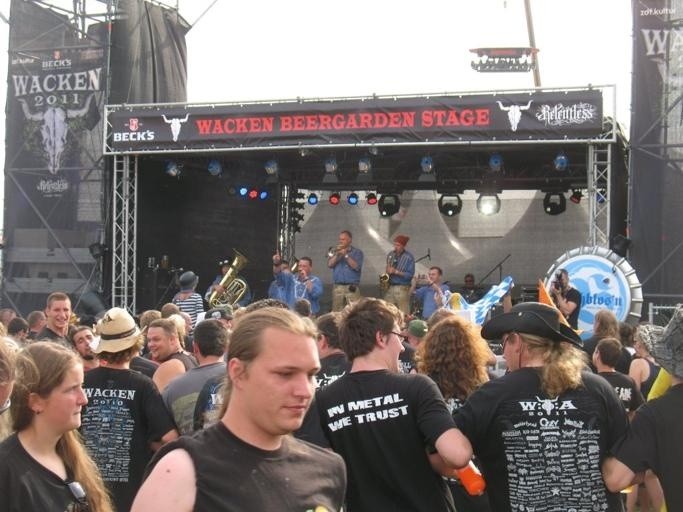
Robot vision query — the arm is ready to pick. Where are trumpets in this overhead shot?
[326,245,346,257]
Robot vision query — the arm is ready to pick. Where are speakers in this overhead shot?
[142,269,184,311]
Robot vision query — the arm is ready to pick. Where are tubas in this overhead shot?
[208,248,250,308]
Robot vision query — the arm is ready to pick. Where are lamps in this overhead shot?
[472,182,505,216]
[166,150,569,180]
[436,184,463,217]
[377,184,403,216]
[568,185,608,206]
[294,186,376,205]
[541,185,569,216]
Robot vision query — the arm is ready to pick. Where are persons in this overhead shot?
[271,245,323,315]
[550,269,580,330]
[499,276,512,314]
[267,261,292,303]
[294,291,683,511]
[130,307,346,511]
[409,266,449,322]
[385,236,414,313]
[204,259,251,307]
[1,291,293,512]
[171,270,203,337]
[460,273,482,304]
[327,230,363,311]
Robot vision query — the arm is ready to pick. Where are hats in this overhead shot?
[407,319,428,338]
[481,302,584,348]
[393,235,409,247]
[638,303,683,379]
[178,271,197,293]
[88,307,148,354]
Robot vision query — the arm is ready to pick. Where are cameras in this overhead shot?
[551,270,562,290]
[418,275,426,278]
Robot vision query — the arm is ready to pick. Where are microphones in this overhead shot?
[428,248,431,260]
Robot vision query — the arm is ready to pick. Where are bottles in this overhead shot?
[456,457,485,497]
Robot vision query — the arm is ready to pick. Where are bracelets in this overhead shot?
[302,276,309,281]
[344,254,348,259]
[272,263,280,267]
[427,281,433,287]
[394,270,398,275]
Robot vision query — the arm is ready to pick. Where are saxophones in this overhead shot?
[379,251,397,291]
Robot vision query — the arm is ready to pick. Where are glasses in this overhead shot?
[382,330,408,344]
